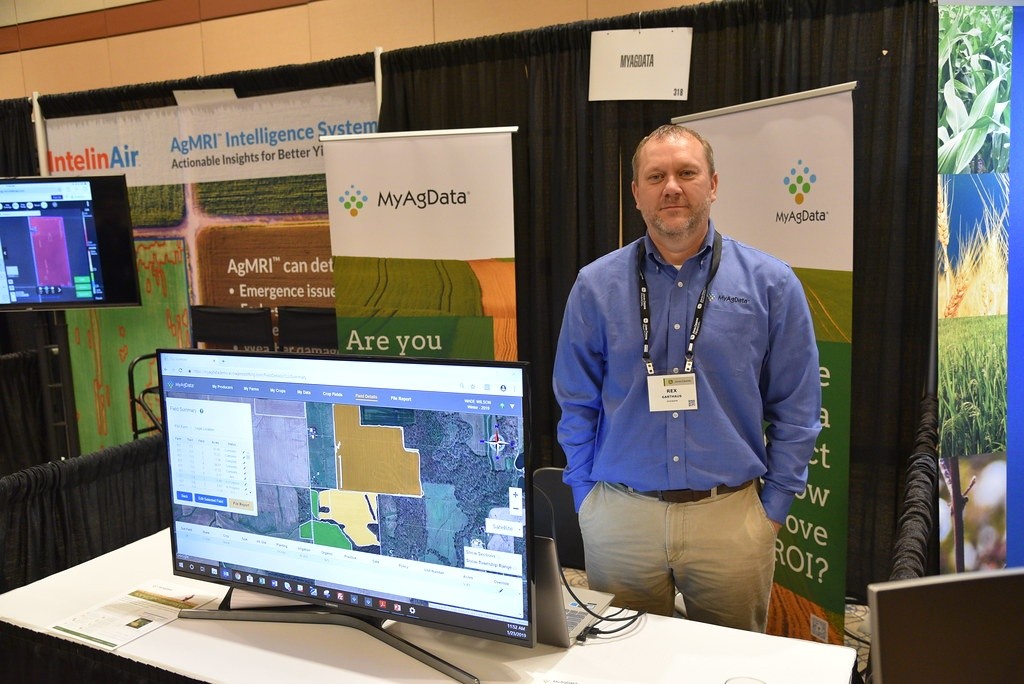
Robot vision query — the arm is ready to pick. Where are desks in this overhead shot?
[0,527,859,684]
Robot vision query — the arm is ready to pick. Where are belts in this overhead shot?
[621,479,755,504]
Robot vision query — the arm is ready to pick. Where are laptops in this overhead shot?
[531,536,616,651]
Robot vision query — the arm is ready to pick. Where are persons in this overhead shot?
[553,124,820,633]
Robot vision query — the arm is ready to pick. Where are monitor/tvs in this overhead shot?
[870,567,1024,684]
[0,175,141,313]
[154,345,534,684]
